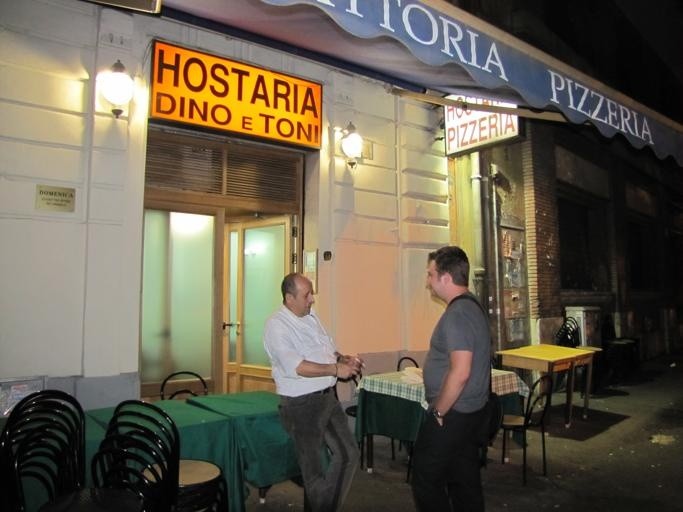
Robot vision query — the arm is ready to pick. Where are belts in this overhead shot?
[312,387,330,395]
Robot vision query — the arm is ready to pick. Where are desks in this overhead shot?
[494,343,596,433]
[186,391,327,504]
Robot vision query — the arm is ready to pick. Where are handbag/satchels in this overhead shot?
[484,390,503,445]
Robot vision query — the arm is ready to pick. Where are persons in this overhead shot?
[260,271,364,511]
[404,245,495,511]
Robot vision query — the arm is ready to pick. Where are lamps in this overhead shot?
[341,121,364,168]
[101,59,135,119]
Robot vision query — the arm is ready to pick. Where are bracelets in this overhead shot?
[333,363,338,377]
[336,354,342,364]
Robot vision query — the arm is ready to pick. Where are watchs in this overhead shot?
[432,407,445,421]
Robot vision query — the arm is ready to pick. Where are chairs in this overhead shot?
[0,371,228,512]
[483,375,553,485]
[554,316,604,398]
[335,356,420,482]
